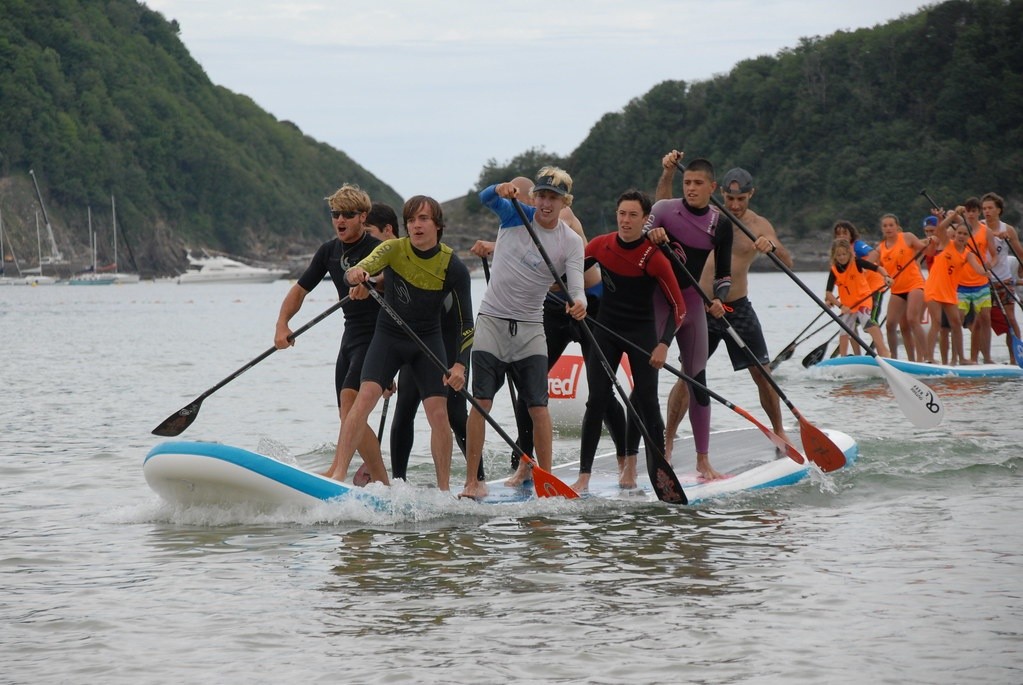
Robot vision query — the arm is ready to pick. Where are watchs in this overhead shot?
[768,241,777,252]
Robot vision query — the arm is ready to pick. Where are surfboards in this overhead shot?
[138,426,857,520]
[815,355,1023,380]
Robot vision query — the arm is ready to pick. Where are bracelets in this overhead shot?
[885,275,891,278]
[839,304,844,308]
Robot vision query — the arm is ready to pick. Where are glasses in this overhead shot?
[329,210,360,219]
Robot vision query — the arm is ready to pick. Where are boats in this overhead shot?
[178,248,291,284]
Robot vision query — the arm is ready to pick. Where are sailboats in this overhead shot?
[0,169,141,286]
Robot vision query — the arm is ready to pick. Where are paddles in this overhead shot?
[865,254,925,356]
[1001,236,1023,269]
[511,185,689,505]
[546,292,806,465]
[776,296,839,362]
[919,188,1023,313]
[769,281,890,371]
[150,294,352,438]
[360,272,581,500]
[665,150,947,432]
[955,210,1023,369]
[481,251,492,285]
[802,237,931,370]
[661,240,847,474]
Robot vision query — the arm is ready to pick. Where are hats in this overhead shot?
[533,175,568,196]
[922,216,937,227]
[722,166,754,193]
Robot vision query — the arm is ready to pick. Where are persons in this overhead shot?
[825,192,1023,365]
[275,149,794,501]
